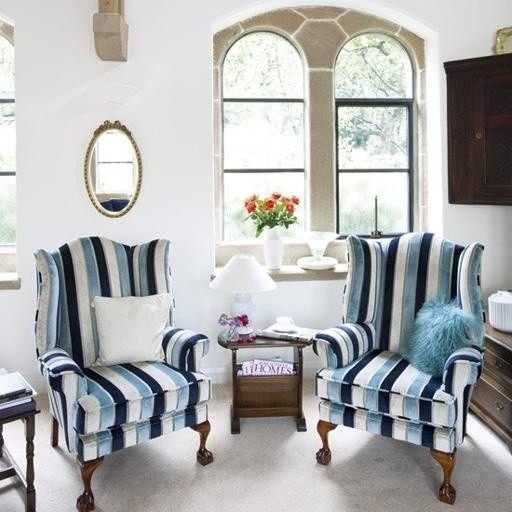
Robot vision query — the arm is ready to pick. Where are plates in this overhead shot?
[273,326,298,333]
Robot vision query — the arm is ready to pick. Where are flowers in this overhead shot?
[241,191,304,239]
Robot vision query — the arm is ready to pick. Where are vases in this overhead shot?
[260,225,285,271]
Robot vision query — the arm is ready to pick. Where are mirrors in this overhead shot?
[83,120,143,219]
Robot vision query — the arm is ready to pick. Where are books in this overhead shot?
[0,371,38,418]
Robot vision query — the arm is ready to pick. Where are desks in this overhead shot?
[0,368,41,511]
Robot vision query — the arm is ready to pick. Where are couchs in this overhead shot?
[310,232,486,504]
[34,237,212,512]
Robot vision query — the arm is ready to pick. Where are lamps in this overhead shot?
[209,254,270,336]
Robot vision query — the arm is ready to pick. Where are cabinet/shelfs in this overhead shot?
[468,303,511,454]
[443,52,512,207]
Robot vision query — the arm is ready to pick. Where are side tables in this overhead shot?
[218,326,314,435]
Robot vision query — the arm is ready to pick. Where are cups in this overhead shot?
[276,316,294,329]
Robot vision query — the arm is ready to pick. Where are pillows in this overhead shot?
[396,293,483,378]
[93,292,171,367]
